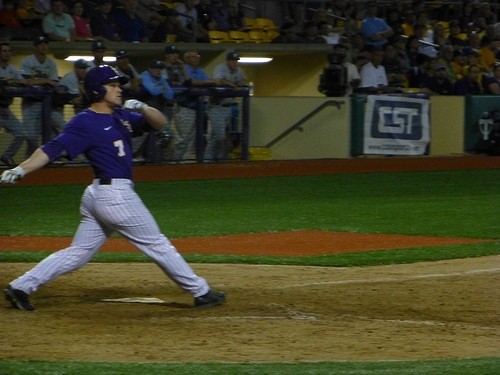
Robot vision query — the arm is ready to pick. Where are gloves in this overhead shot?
[0,166,26,184]
[123,99,148,112]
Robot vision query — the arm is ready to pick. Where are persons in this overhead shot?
[0,65,227,312]
[0,0,500,169]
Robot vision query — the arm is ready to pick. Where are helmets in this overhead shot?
[84,66,129,103]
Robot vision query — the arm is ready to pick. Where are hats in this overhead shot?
[435,65,446,71]
[166,44,181,54]
[148,56,166,69]
[493,62,500,68]
[92,42,107,51]
[34,36,49,45]
[226,52,240,61]
[116,50,129,58]
[74,60,91,68]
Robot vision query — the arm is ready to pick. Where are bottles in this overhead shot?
[249,82,254,96]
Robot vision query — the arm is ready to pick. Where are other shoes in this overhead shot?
[194,289,226,309]
[3,285,34,312]
[0,157,19,166]
